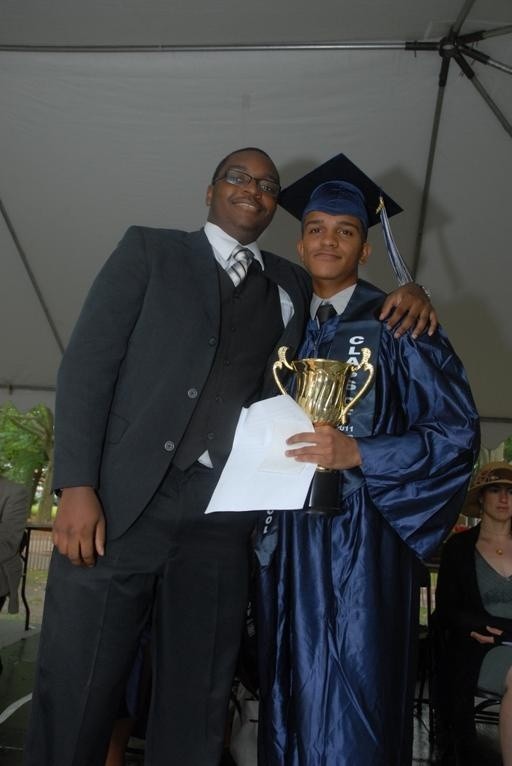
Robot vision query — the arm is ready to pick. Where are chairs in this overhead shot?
[0,527,32,631]
[416,567,503,732]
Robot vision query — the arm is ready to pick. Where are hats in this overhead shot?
[460,462,511,519]
[276,151,405,237]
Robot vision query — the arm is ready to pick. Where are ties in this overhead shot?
[313,302,337,357]
[228,245,249,288]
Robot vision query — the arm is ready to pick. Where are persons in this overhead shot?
[0,472,30,615]
[238,153,480,766]
[425,461,512,766]
[28,147,439,766]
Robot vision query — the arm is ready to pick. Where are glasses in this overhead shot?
[210,168,280,195]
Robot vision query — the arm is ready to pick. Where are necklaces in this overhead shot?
[495,548,504,555]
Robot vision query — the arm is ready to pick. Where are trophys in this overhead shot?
[273,345,376,515]
